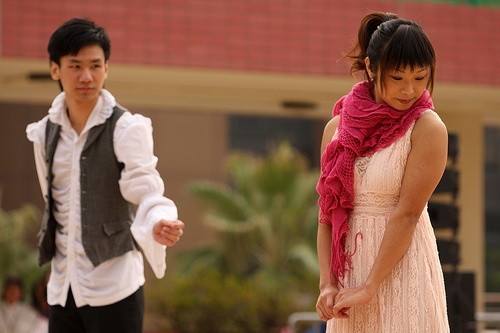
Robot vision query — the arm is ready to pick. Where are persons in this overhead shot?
[0,274,52,333]
[24,16,184,333]
[315,11,451,333]
